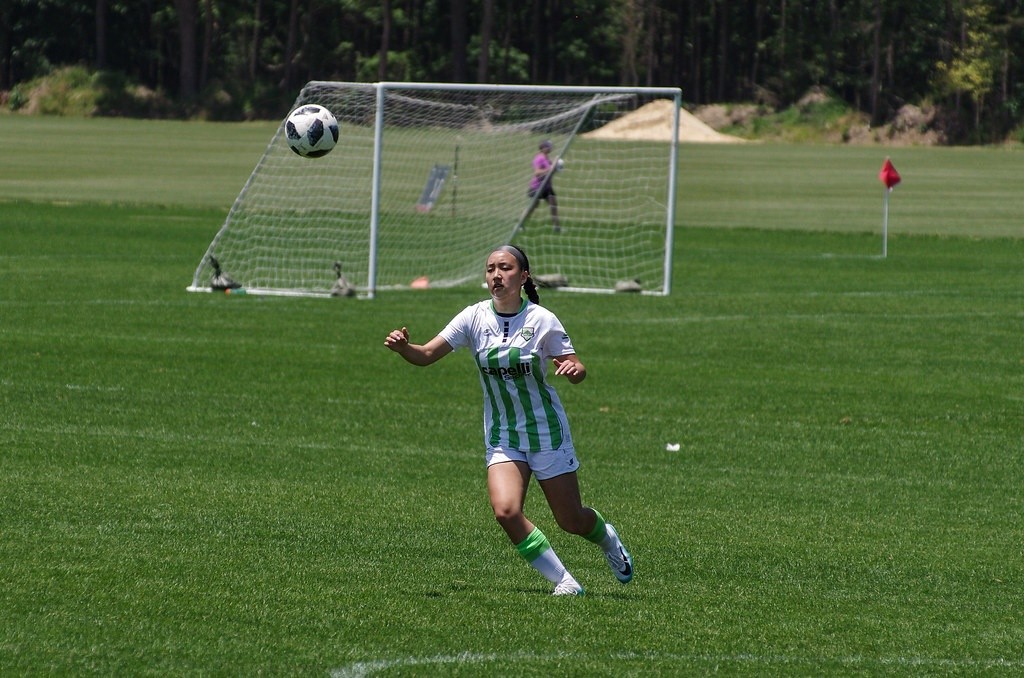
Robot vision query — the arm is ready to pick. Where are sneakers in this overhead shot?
[550,581,585,597]
[599,524,634,584]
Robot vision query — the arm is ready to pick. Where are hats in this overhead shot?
[539,140,553,150]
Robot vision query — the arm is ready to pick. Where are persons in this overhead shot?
[518,140,565,234]
[383,244,633,595]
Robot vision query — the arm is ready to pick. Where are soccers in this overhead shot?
[285,103,339,159]
